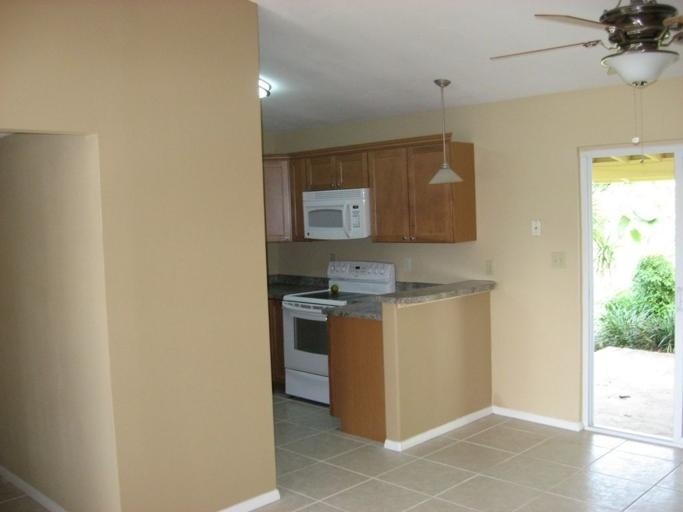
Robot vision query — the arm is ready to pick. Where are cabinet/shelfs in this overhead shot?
[371,142,477,244]
[264,154,300,243]
[306,151,369,191]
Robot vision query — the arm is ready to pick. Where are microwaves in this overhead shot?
[301,187,370,242]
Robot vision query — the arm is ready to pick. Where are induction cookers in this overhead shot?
[282,289,376,307]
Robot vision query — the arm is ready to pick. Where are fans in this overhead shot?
[489,0,683,87]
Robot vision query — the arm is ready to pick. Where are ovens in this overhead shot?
[281,300,333,407]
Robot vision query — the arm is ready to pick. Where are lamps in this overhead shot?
[601,45,679,87]
[256,77,273,100]
[428,78,465,186]
